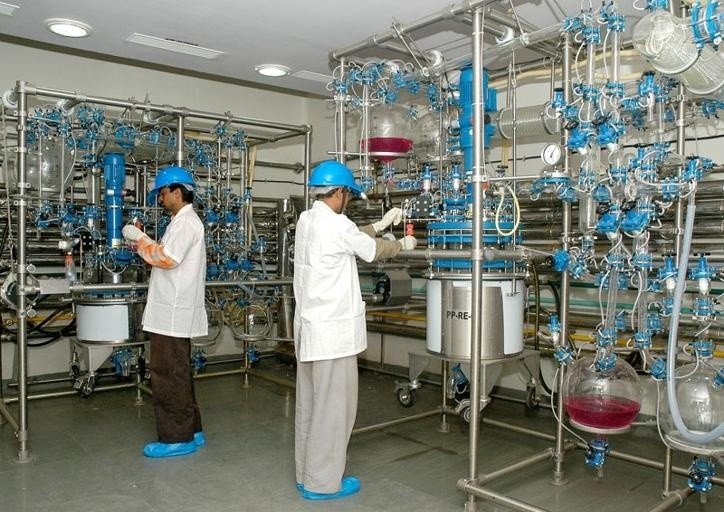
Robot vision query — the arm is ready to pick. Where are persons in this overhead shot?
[122,168,209,457]
[290,164,417,501]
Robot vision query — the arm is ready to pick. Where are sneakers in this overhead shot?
[193,432,208,450]
[141,437,198,458]
[302,475,361,501]
[295,482,303,493]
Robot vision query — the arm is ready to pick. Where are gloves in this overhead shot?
[121,224,146,243]
[371,206,402,234]
[398,235,418,251]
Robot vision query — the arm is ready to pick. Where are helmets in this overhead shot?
[303,159,364,195]
[151,166,197,196]
